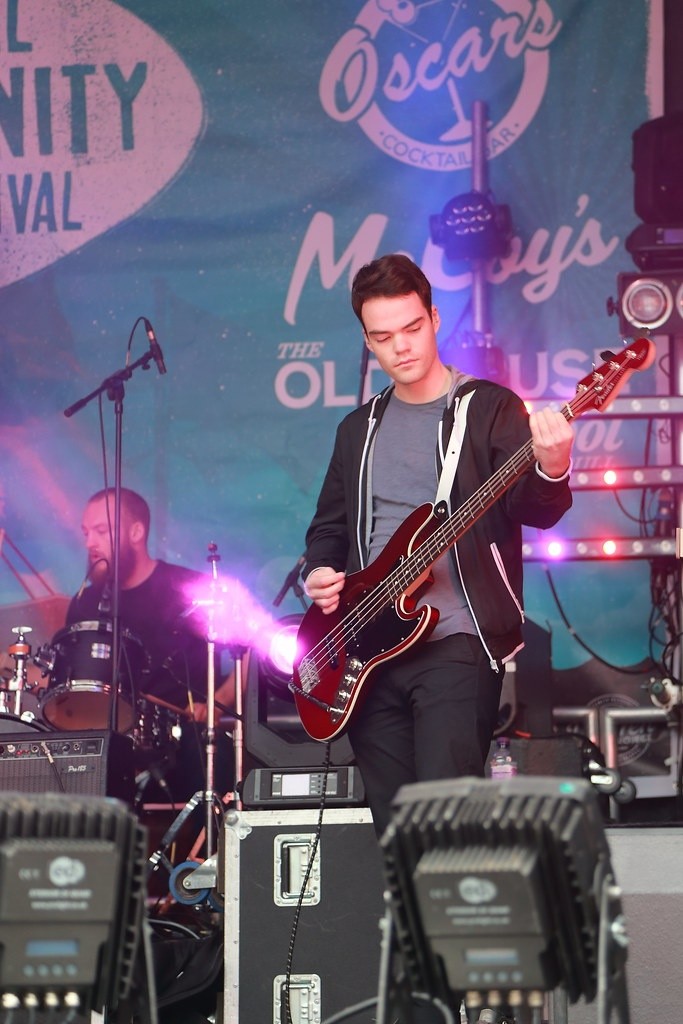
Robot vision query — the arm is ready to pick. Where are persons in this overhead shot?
[301,255,574,840]
[50,487,251,803]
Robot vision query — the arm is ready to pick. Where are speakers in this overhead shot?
[491,617,553,737]
[0,729,135,811]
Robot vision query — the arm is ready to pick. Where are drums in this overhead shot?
[39,618,148,734]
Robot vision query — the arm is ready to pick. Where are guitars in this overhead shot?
[291,334,657,745]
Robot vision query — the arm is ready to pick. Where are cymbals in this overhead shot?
[1,650,50,698]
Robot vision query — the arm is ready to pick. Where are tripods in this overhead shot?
[148,542,245,874]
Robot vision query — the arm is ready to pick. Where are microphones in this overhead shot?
[98,574,113,612]
[273,553,306,607]
[143,319,166,374]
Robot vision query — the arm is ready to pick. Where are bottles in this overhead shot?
[490,737,518,780]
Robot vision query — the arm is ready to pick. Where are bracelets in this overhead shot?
[214,698,226,713]
